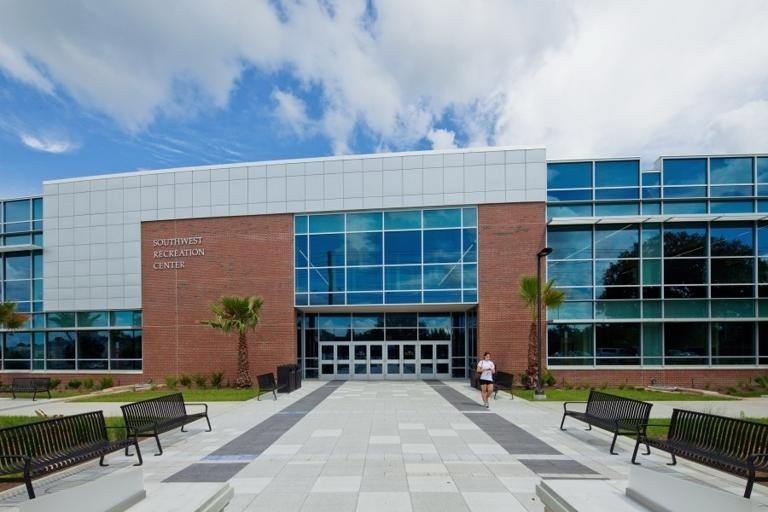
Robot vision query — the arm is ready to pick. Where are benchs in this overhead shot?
[560,391,768,499]
[492,371,515,400]
[256,372,288,401]
[0,377,212,499]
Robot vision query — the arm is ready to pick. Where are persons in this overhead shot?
[476,352,495,408]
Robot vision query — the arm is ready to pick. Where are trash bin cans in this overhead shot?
[277,364,301,393]
[469,368,482,390]
